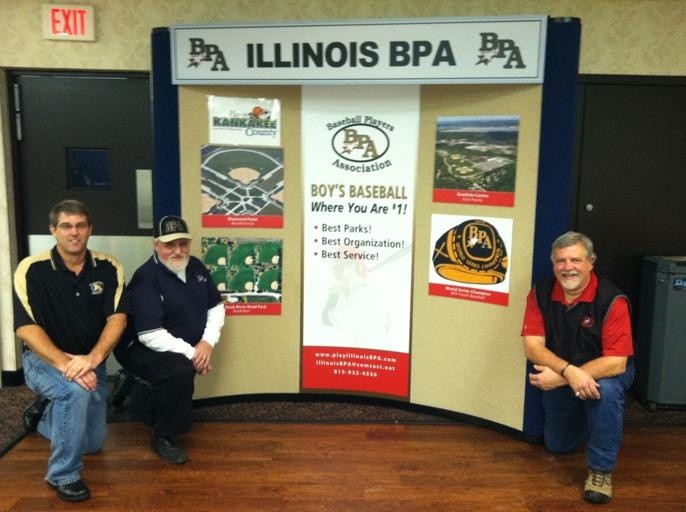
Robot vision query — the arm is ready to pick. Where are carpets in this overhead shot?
[0,380,41,456]
[107,373,686,425]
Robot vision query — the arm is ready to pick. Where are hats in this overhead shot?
[153,215,193,242]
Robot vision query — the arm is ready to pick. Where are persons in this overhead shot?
[108,213,227,466]
[14,199,127,501]
[520,231,636,502]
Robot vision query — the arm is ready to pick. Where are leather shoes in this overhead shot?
[150,432,189,464]
[23,395,49,429]
[46,478,90,502]
[107,368,131,413]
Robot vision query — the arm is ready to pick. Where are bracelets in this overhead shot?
[561,363,571,375]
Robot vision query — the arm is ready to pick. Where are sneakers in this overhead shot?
[583,469,613,504]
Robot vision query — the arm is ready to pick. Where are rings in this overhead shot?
[574,392,579,398]
